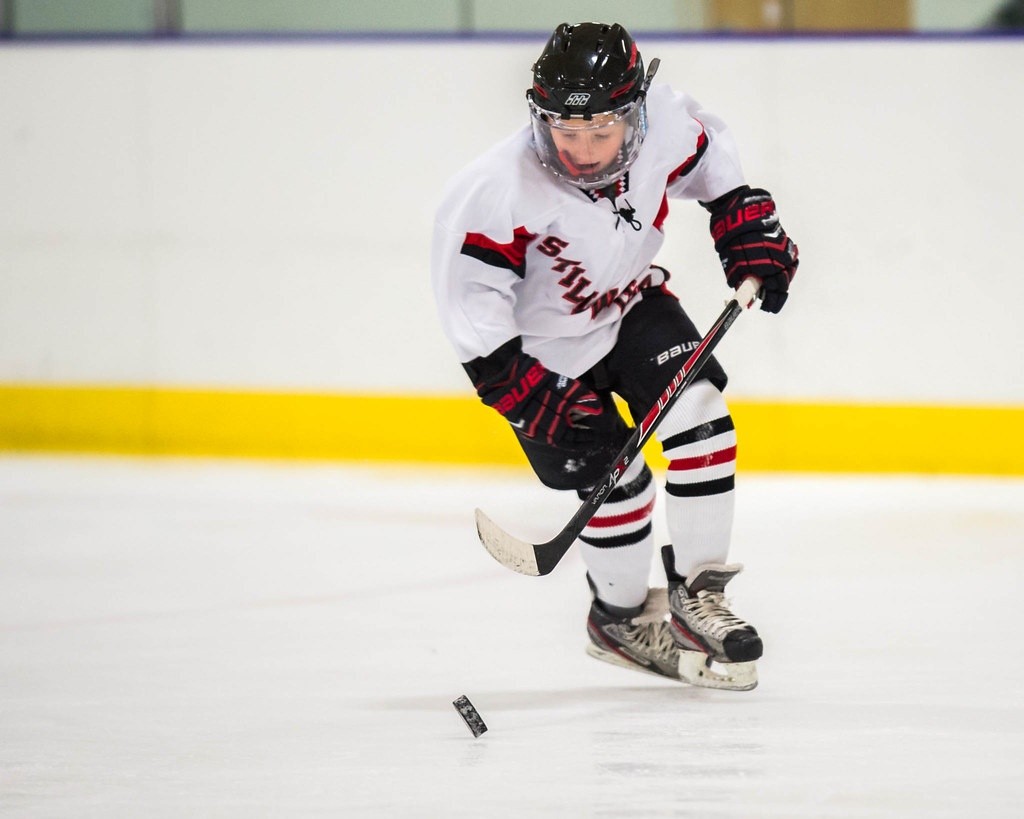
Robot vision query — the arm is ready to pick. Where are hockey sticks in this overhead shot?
[476,271,761,575]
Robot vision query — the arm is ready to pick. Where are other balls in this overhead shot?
[452,692,490,740]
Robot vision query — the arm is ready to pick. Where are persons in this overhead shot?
[438,20,800,692]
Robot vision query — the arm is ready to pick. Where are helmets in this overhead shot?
[526,22,661,190]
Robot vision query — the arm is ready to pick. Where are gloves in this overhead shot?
[709,188,799,314]
[477,353,603,452]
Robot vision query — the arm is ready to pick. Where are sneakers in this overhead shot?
[585,569,680,681]
[661,545,762,691]
